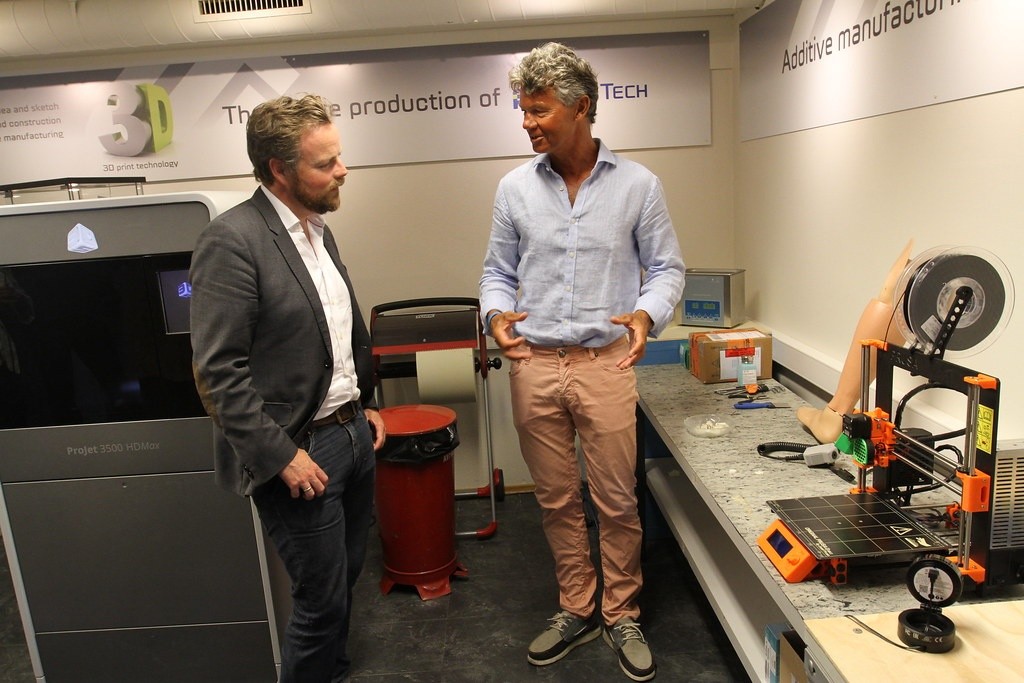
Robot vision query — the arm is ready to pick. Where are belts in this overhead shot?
[309,399,362,430]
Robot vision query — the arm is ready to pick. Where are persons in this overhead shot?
[479,41,685,683]
[189,93,388,683]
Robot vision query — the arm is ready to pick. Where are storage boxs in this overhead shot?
[780,630,808,683]
[688,327,775,384]
[674,267,747,328]
[679,342,690,369]
[763,622,791,683]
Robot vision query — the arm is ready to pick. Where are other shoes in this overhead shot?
[330,659,350,683]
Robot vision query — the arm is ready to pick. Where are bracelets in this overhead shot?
[488,312,500,325]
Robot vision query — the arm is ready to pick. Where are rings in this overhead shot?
[303,487,311,492]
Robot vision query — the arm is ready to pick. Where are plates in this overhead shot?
[684,414,735,437]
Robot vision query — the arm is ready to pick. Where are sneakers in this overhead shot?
[602,616,656,681]
[527,606,602,664]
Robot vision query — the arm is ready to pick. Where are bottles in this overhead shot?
[737,356,758,386]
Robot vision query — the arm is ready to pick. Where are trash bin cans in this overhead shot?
[372,403,469,602]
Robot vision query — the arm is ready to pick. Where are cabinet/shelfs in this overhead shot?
[631,362,1024,683]
